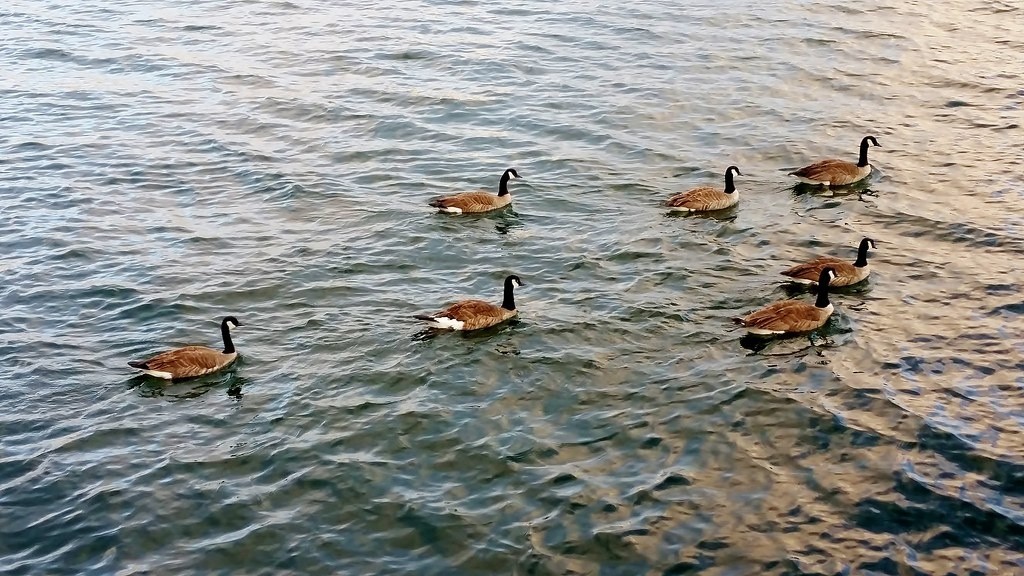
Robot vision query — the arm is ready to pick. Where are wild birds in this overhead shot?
[664,165,745,214]
[410,275,528,345]
[127,316,246,380]
[428,168,523,214]
[787,135,883,186]
[741,238,877,333]
